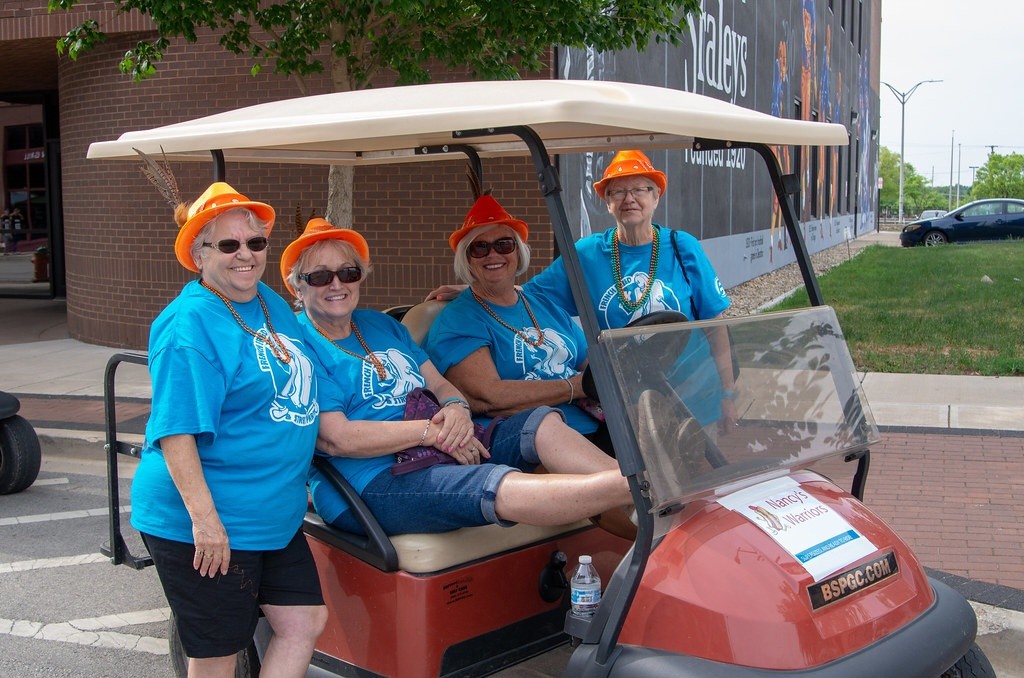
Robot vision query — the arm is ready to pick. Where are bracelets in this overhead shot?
[721,389,738,400]
[419,418,430,446]
[565,378,574,404]
[440,396,471,406]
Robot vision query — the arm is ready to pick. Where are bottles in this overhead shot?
[570,555,602,617]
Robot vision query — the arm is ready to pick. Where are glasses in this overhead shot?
[470,237,517,258]
[608,187,653,200]
[299,267,361,287]
[203,237,269,253]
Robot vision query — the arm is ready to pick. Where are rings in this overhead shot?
[196,550,204,557]
[206,553,214,558]
[736,423,739,426]
[470,447,478,451]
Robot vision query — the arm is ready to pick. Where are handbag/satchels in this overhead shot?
[390,387,505,476]
[670,230,740,384]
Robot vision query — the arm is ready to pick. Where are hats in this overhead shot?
[593,150,667,201]
[280,217,369,297]
[450,193,529,253]
[174,181,276,273]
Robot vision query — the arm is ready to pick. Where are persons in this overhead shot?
[279,216,707,539]
[421,194,639,542]
[424,149,737,452]
[129,184,328,678]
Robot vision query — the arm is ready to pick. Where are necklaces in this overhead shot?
[313,320,386,382]
[202,279,291,364]
[610,225,660,311]
[470,288,543,346]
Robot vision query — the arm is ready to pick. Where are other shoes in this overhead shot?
[677,417,715,497]
[638,390,690,511]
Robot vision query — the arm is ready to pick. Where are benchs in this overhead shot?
[303,299,597,577]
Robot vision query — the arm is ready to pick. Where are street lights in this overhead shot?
[969,166,979,181]
[880,80,943,224]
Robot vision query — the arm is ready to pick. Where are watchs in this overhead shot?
[443,400,473,418]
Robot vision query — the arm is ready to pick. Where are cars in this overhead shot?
[917,210,947,220]
[899,198,1024,247]
